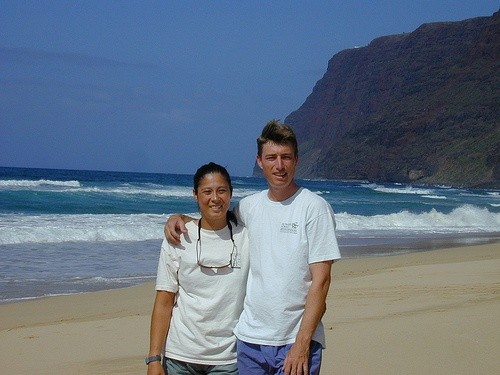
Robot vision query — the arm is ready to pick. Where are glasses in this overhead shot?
[196,239,234,268]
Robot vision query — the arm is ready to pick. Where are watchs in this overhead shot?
[145,355,161,364]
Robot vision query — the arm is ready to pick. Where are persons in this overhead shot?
[163,119,342,375]
[145,161,249,375]
